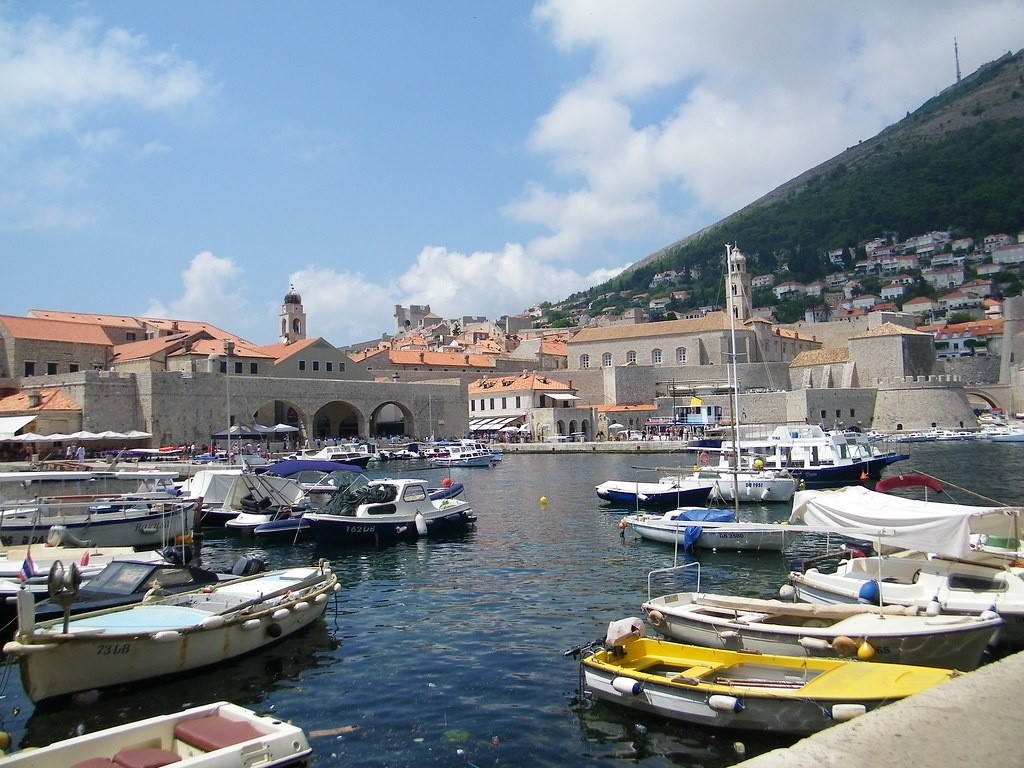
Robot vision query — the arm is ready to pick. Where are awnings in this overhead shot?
[0,415,38,438]
[546,393,582,401]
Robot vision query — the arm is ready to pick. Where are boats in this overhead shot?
[877,474,1024,574]
[578,618,970,731]
[621,504,802,550]
[643,562,1005,672]
[1,699,313,768]
[784,550,1024,644]
[0,438,500,699]
[595,478,713,505]
[687,423,910,482]
[866,412,1024,445]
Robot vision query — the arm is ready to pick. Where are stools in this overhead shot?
[115,747,182,768]
[69,756,121,768]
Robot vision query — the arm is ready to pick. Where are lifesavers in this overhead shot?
[699,452,710,464]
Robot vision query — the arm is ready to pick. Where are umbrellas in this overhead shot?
[499,426,530,435]
[609,424,624,432]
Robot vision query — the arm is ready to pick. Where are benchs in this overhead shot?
[175,714,269,755]
[735,616,761,622]
[681,666,713,680]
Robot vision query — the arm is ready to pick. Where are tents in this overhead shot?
[0,430,153,450]
[211,421,300,452]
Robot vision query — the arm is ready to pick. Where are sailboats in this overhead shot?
[658,244,797,504]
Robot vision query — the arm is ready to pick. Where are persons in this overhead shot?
[181,434,368,460]
[371,432,530,444]
[596,430,604,443]
[610,431,613,441]
[65,442,87,464]
[627,427,631,440]
[641,425,702,442]
